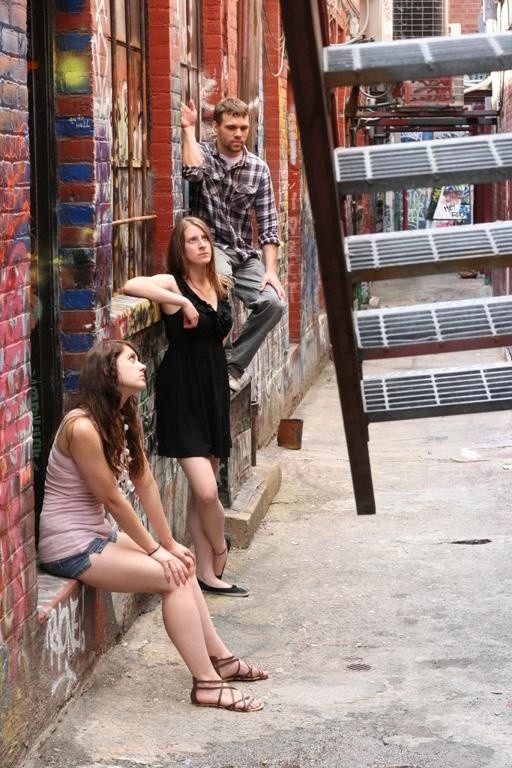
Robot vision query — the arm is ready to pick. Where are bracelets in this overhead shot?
[148,545,164,555]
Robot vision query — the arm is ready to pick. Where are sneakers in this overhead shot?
[228,373,240,392]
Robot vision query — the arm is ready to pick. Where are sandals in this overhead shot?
[191,676,264,712]
[210,656,270,683]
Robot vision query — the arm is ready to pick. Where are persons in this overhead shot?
[117,214,247,597]
[180,91,286,397]
[36,335,267,713]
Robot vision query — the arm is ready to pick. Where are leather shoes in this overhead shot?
[197,577,249,596]
[216,534,231,579]
